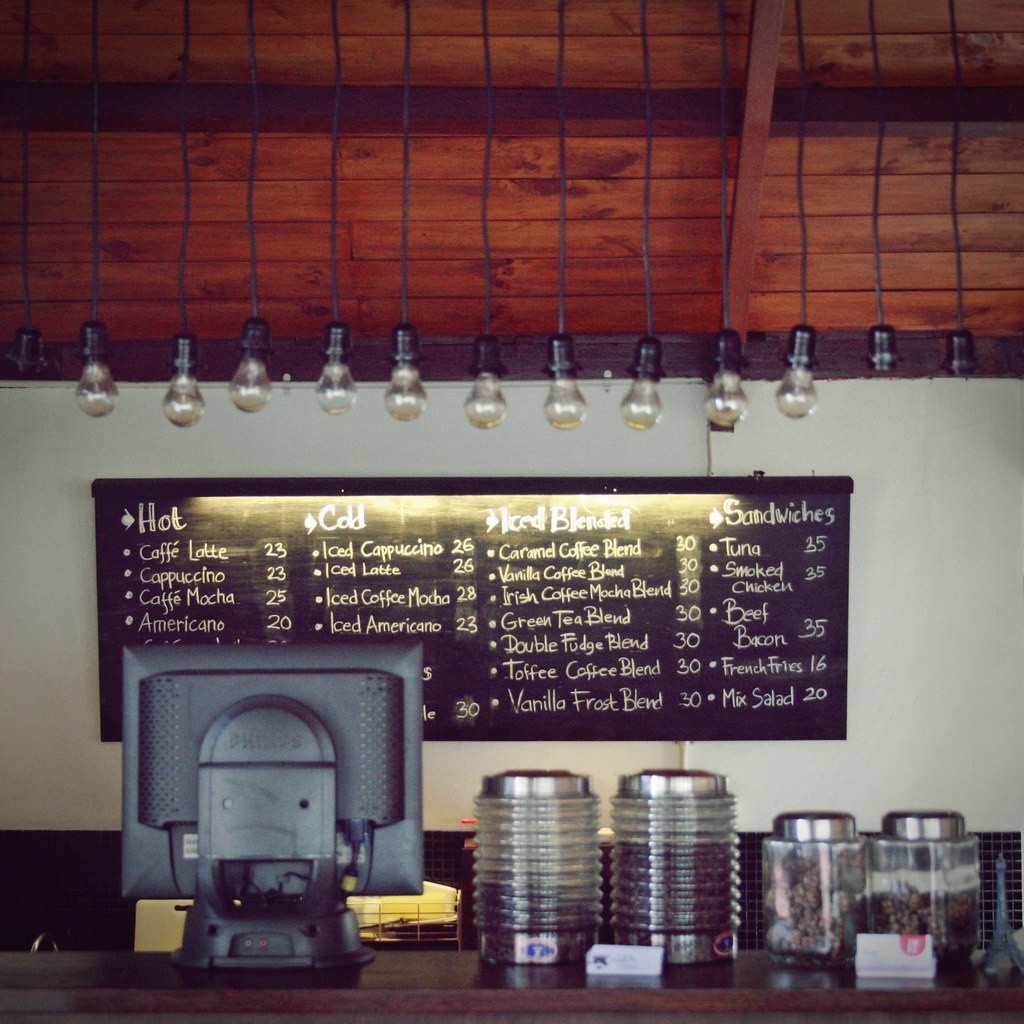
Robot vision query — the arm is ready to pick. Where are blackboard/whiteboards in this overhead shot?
[90,474,854,741]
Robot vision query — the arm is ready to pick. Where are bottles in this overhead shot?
[470,771,602,966]
[611,770,740,965]
[762,810,866,967]
[869,811,980,972]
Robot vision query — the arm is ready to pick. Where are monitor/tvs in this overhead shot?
[121,638,423,991]
